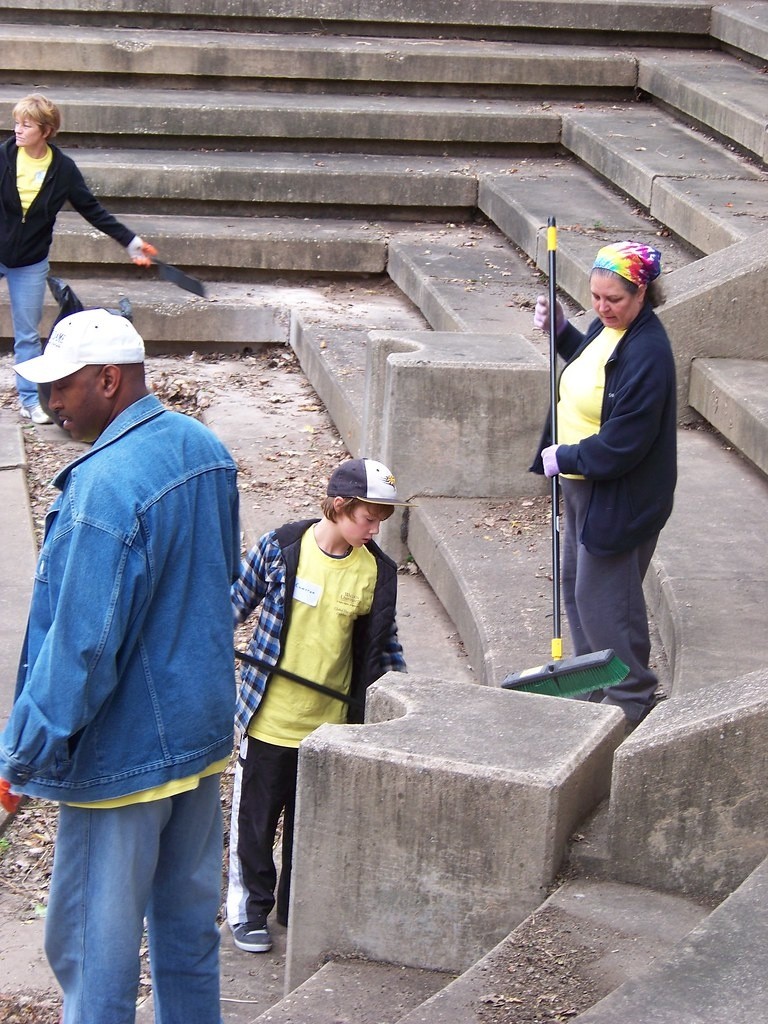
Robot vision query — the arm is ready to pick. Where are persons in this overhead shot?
[229,457,415,953]
[0,91,157,425]
[526,241,681,736]
[0,311,240,1024]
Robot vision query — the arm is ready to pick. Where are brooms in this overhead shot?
[504,216,633,695]
[233,648,365,709]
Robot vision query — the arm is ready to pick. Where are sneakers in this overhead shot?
[230,921,272,952]
[20,404,54,424]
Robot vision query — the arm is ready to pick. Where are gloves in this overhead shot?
[541,444,561,479]
[533,295,566,334]
[126,235,158,267]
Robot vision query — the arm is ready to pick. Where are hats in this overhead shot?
[593,240,662,285]
[12,308,146,383]
[327,458,420,507]
